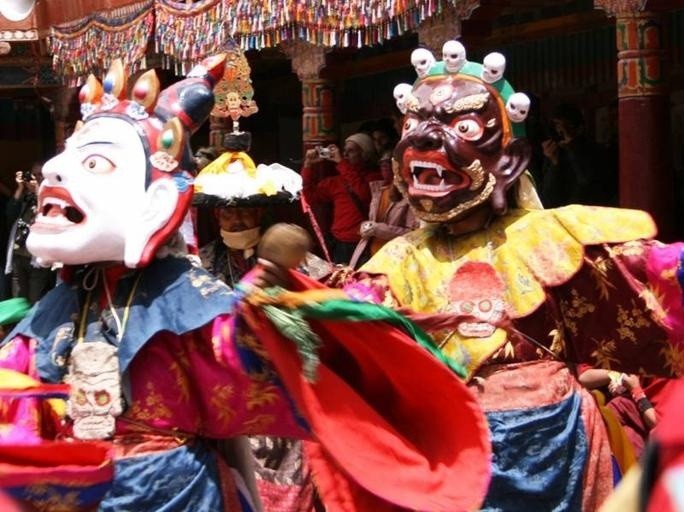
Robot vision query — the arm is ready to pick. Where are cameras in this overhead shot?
[22,168,36,183]
[318,147,331,159]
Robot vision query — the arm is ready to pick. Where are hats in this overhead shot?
[344,133,379,163]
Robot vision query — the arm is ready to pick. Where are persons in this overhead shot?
[4,163,54,306]
[522,91,619,208]
[1,56,492,511]
[190,132,338,512]
[301,108,403,265]
[354,37,684,511]
